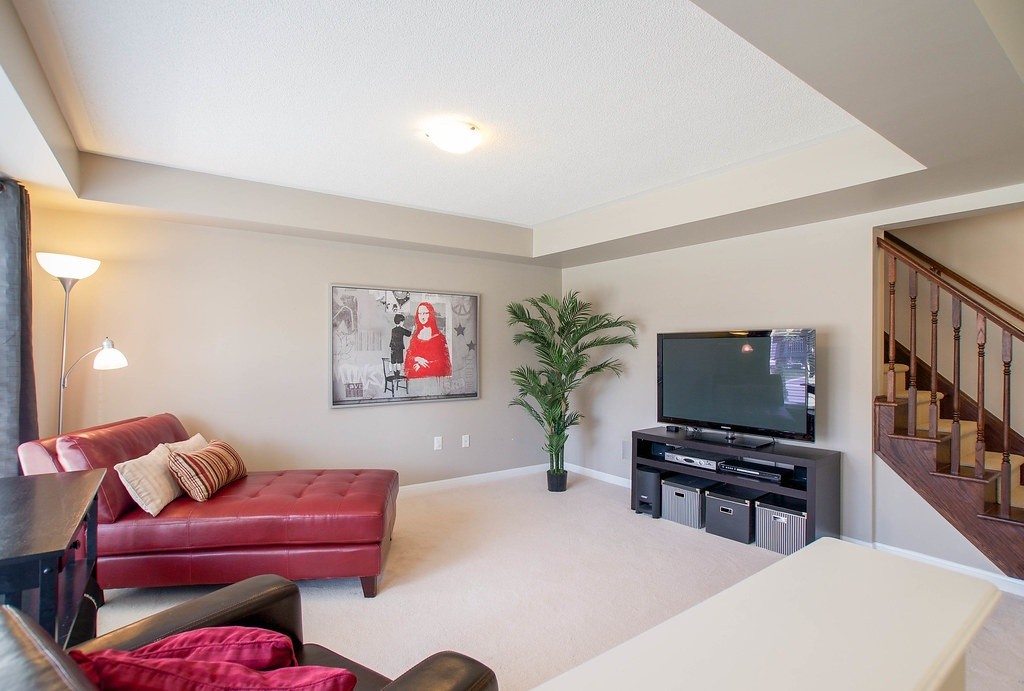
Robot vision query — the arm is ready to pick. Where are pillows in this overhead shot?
[67,625,357,690]
[55,412,191,525]
[114,443,185,516]
[164,432,208,455]
[167,439,248,502]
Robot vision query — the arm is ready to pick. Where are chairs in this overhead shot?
[0,573,499,691]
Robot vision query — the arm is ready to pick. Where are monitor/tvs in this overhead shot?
[656,328,817,449]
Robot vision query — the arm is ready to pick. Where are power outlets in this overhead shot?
[434,436,443,450]
[462,434,469,447]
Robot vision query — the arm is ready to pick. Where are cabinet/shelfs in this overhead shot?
[631,426,840,554]
[0,467,108,649]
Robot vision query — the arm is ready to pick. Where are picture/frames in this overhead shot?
[329,282,480,408]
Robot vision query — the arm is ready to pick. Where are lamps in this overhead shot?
[35,251,128,434]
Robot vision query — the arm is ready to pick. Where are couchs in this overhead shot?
[18,415,399,598]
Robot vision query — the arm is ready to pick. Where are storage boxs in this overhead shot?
[705,483,769,544]
[754,492,808,555]
[661,473,725,528]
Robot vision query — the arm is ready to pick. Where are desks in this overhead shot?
[532,534,1003,690]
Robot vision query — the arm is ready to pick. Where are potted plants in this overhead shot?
[504,290,639,491]
